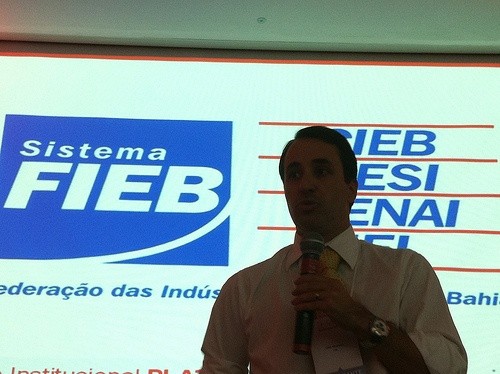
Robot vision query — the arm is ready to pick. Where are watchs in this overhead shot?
[366,316,390,346]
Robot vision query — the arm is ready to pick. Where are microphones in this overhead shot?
[292,232,324,355]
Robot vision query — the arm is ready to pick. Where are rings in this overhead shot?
[314,292,319,301]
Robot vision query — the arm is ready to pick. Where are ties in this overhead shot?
[318,246,361,374]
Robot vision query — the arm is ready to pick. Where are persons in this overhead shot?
[201,125,469,374]
